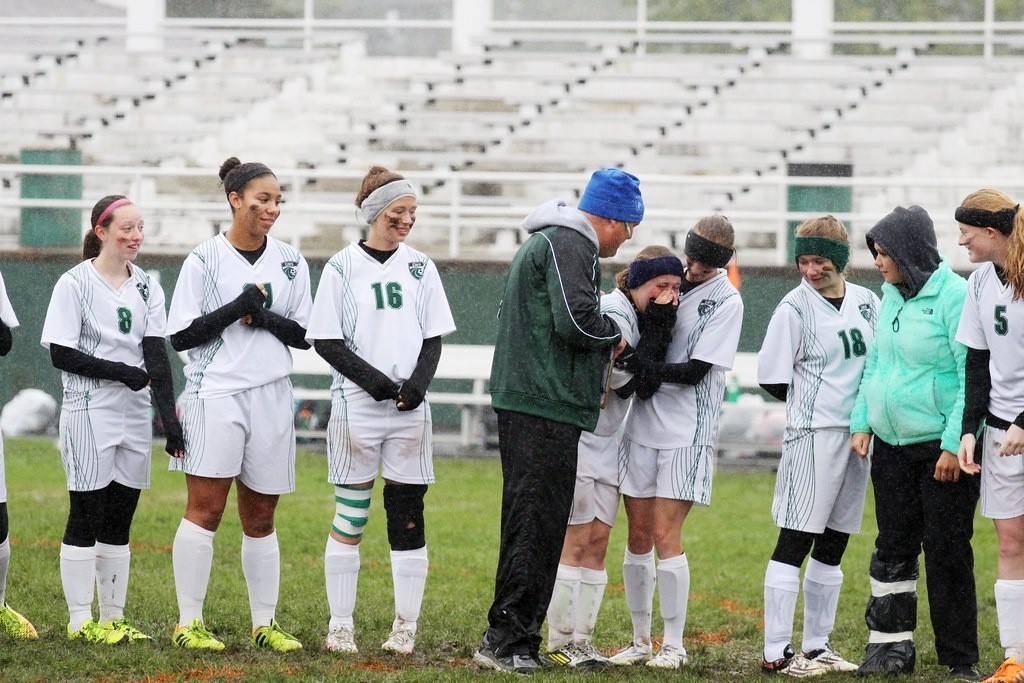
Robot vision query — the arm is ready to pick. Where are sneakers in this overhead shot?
[979,658,1024,683]
[533,654,564,671]
[801,642,858,671]
[104,618,152,642]
[544,640,606,672]
[327,626,359,654]
[760,644,825,678]
[0,603,39,640]
[67,619,129,645]
[948,664,979,680]
[252,618,303,653]
[610,641,652,665]
[172,618,225,650]
[473,632,542,678]
[644,640,689,668]
[578,641,616,669]
[381,625,415,654]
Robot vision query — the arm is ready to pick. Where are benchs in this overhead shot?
[290,340,494,450]
[0,28,1024,267]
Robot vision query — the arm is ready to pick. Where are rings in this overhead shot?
[942,473,947,476]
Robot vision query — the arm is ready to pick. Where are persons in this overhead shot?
[953,188,1024,683]
[469,171,644,677]
[1,272,39,641]
[169,156,314,651]
[757,214,882,678]
[39,194,185,644]
[305,166,456,656]
[847,205,982,679]
[544,245,682,669]
[611,213,743,671]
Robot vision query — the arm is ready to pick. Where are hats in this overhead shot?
[578,167,645,223]
